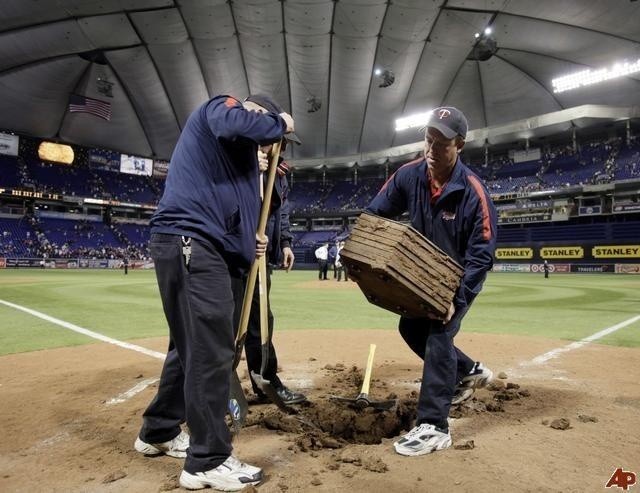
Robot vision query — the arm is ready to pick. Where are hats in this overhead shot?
[418,105,469,140]
[244,93,302,146]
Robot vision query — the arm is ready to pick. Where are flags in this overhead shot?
[68,90,113,122]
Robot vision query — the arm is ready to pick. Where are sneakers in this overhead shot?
[178,455,265,492]
[450,360,494,405]
[133,429,192,458]
[256,385,307,405]
[393,422,453,457]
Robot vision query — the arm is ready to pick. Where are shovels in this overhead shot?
[227,135,284,439]
[250,146,298,415]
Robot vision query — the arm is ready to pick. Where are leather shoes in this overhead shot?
[319,274,351,281]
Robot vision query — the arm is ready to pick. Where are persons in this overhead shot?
[122,255,129,274]
[327,239,342,280]
[287,177,385,213]
[132,92,295,488]
[363,104,498,459]
[314,240,330,280]
[543,258,550,278]
[0,134,168,268]
[334,241,347,282]
[468,127,640,194]
[231,129,307,410]
